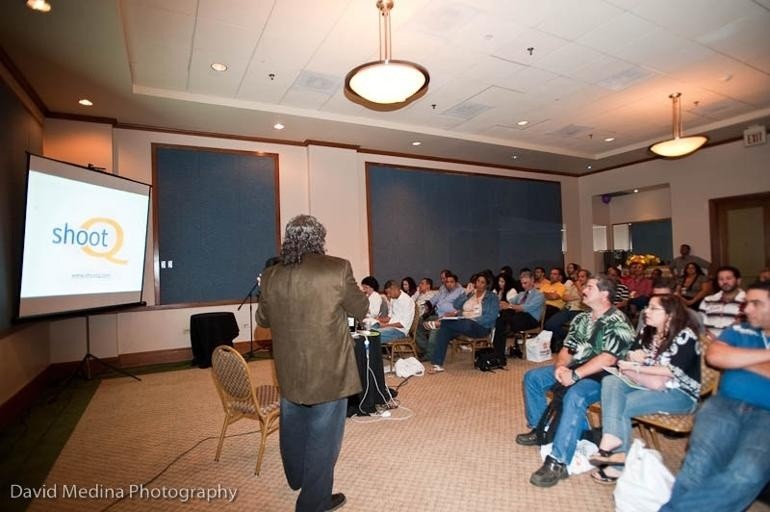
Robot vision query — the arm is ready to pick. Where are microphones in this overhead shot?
[257,272,263,283]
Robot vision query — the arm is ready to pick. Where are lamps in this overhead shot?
[649,92,707,158]
[346,0,431,113]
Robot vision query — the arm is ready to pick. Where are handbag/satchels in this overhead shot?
[395,357,425,378]
[536,397,563,445]
[475,347,503,371]
[526,330,553,362]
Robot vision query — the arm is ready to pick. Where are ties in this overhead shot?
[519,291,528,305]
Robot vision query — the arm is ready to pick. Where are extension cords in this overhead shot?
[370,411,391,418]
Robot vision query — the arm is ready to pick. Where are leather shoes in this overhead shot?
[516,428,548,445]
[326,493,345,512]
[530,455,569,487]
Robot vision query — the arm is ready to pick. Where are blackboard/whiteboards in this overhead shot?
[367,162,568,292]
[151,143,280,308]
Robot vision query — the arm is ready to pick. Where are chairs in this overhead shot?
[546,329,721,451]
[211,345,280,476]
[381,298,546,375]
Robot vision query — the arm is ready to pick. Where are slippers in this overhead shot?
[429,364,444,373]
[590,449,625,465]
[423,321,439,330]
[591,468,620,485]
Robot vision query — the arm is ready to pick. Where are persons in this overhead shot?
[356,244,770,512]
[255,213,369,511]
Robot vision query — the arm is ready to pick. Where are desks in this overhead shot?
[346,329,398,418]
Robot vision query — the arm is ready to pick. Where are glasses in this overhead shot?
[644,305,666,312]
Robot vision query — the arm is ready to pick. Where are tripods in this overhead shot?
[241,295,273,364]
